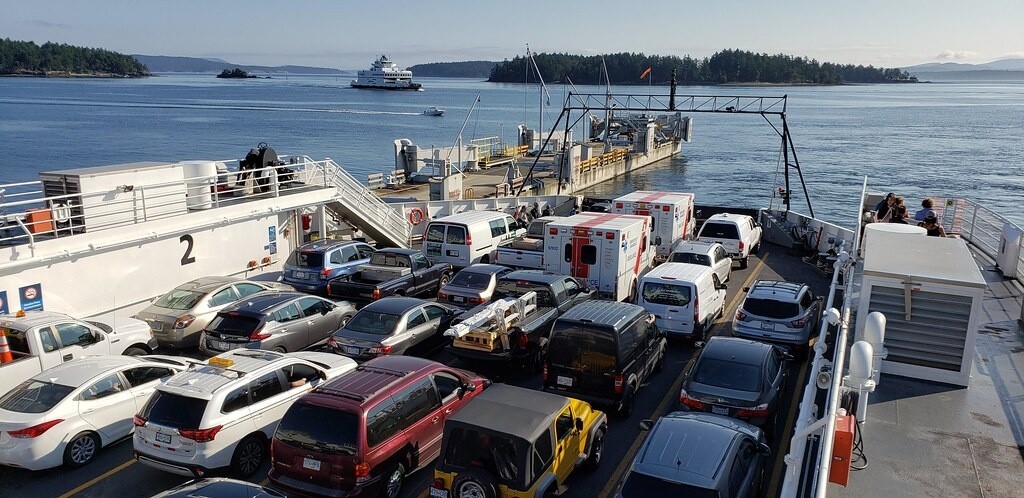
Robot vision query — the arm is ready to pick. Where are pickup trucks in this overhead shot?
[449,268,600,369]
[492,214,569,270]
[326,246,454,311]
[0,308,159,400]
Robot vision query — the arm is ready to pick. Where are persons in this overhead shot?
[875,193,947,237]
[518,203,554,224]
[570,205,580,216]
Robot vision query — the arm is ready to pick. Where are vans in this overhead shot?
[542,297,668,419]
[421,209,528,273]
[635,260,728,349]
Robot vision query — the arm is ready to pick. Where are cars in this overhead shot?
[0,354,203,472]
[326,295,469,366]
[146,477,293,498]
[613,409,769,498]
[437,261,517,311]
[664,241,734,285]
[677,335,796,442]
[198,290,358,363]
[589,202,612,212]
[128,274,298,354]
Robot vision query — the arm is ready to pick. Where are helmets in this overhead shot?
[546,205,551,209]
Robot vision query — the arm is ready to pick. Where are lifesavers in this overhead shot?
[409,208,424,225]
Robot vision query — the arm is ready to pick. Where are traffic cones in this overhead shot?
[0,329,13,365]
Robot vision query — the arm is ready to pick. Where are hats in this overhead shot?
[925,210,937,219]
[896,205,906,214]
[895,198,902,204]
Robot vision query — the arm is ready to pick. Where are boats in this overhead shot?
[424,106,446,116]
[349,53,424,91]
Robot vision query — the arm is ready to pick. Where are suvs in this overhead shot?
[695,211,764,269]
[428,381,609,498]
[730,278,824,363]
[280,236,382,298]
[130,346,359,484]
[267,352,493,498]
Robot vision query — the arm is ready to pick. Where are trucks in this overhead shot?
[610,188,697,261]
[542,211,663,303]
